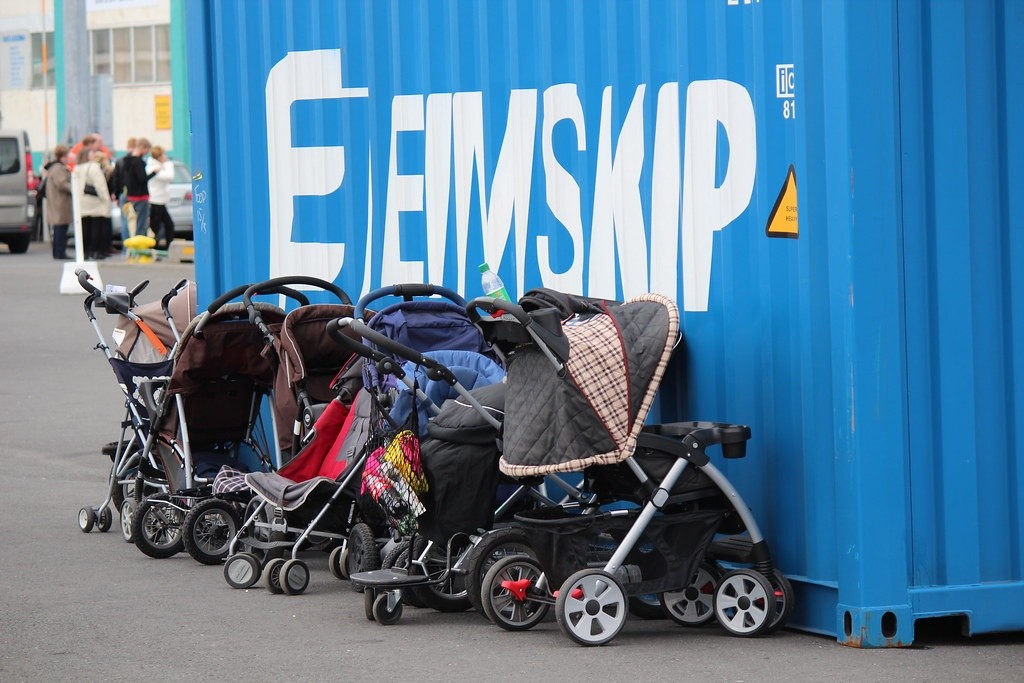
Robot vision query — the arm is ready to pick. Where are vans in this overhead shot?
[1,127,36,256]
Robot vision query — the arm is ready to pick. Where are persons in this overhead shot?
[44,145,72,259]
[69,134,137,258]
[123,138,161,237]
[145,147,175,248]
[72,150,112,260]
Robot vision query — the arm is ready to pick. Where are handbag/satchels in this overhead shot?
[84,163,97,195]
[37,176,48,197]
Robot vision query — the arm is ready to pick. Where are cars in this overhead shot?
[38,158,193,248]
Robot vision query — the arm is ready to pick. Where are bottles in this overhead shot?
[478,263,512,303]
[361,471,407,518]
[377,457,428,519]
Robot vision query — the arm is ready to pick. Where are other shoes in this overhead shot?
[54,254,71,259]
[85,248,115,259]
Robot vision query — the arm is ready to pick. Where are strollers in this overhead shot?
[75,268,797,645]
